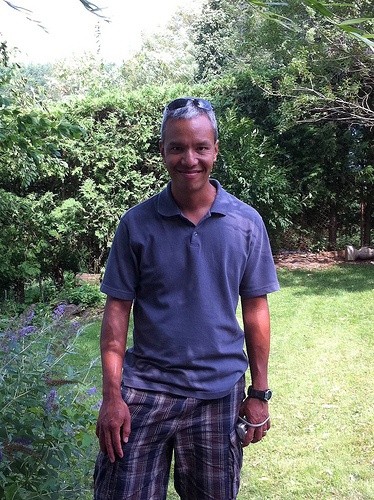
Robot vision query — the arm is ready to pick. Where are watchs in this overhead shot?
[247,384,273,402]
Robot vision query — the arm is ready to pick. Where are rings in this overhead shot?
[263,430,267,433]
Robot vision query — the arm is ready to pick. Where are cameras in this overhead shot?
[236,419,247,440]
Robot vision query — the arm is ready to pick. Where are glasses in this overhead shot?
[167,98,214,110]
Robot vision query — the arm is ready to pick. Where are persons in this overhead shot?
[92,96,281,500]
